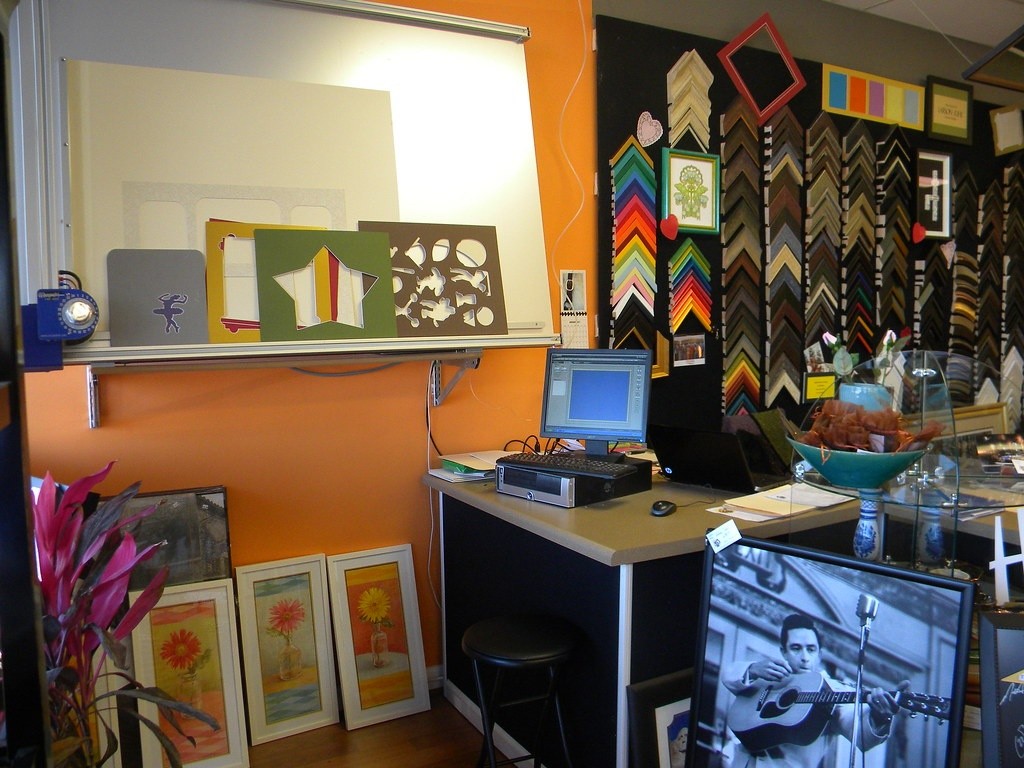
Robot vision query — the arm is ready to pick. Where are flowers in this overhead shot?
[822,329,912,386]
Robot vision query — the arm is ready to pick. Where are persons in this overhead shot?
[722,613,911,767]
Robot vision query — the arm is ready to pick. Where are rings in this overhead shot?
[887,718,891,721]
[874,701,880,705]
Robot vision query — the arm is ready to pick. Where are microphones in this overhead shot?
[856,594,878,661]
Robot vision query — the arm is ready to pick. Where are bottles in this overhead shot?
[917,505,944,566]
[852,488,884,562]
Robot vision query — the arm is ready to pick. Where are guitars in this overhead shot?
[725,670,952,753]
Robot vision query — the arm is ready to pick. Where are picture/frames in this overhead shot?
[979,602,1024,768]
[914,146,953,238]
[128,577,250,768]
[233,554,340,747]
[626,664,696,767]
[327,543,431,731]
[924,75,973,145]
[661,147,720,234]
[903,401,1010,457]
[684,528,974,768]
[96,485,232,585]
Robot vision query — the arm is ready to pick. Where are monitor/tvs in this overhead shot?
[538,347,653,464]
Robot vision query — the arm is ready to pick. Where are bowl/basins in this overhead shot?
[784,430,935,491]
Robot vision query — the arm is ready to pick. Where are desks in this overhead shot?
[420,451,861,768]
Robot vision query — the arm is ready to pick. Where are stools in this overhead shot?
[461,609,573,768]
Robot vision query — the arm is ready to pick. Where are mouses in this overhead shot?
[652,500,678,517]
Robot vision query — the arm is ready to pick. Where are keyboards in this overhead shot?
[495,452,638,481]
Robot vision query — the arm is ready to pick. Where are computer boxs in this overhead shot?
[497,456,654,508]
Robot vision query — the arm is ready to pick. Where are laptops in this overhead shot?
[649,422,796,493]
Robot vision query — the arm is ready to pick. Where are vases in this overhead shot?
[838,380,893,412]
